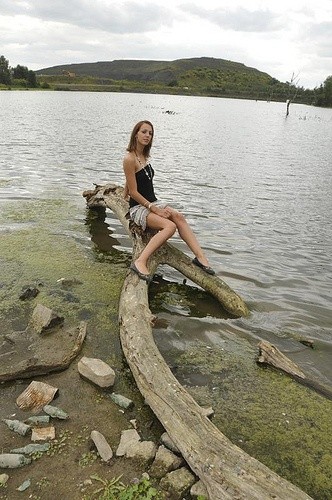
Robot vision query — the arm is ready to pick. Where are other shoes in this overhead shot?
[131,263,153,282]
[192,257,215,275]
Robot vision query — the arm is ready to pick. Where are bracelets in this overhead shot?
[144,201,153,209]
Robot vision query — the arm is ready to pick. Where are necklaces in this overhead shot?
[134,148,153,181]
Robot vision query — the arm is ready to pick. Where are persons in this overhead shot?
[123,120,216,281]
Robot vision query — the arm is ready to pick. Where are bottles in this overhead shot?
[22,416,50,424]
[0,453,31,468]
[159,432,181,453]
[43,405,69,420]
[107,393,134,409]
[1,417,31,436]
